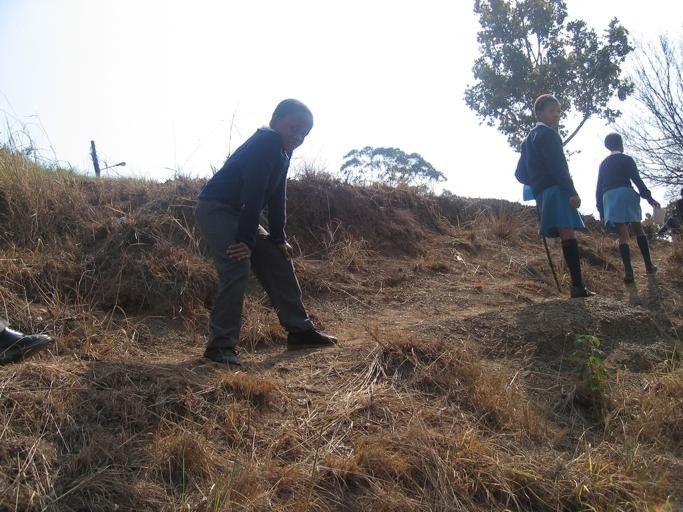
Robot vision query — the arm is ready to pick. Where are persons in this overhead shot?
[595,133,660,283]
[0,315,55,364]
[193,98,338,368]
[513,94,596,298]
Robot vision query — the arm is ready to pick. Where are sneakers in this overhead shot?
[200,345,244,370]
[0,325,53,366]
[569,287,597,298]
[646,264,659,273]
[283,327,339,350]
[622,275,637,284]
